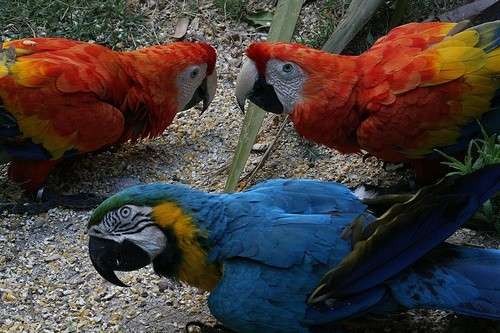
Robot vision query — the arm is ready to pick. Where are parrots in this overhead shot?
[236,20,500,176]
[0,37,217,215]
[87,178,500,333]
